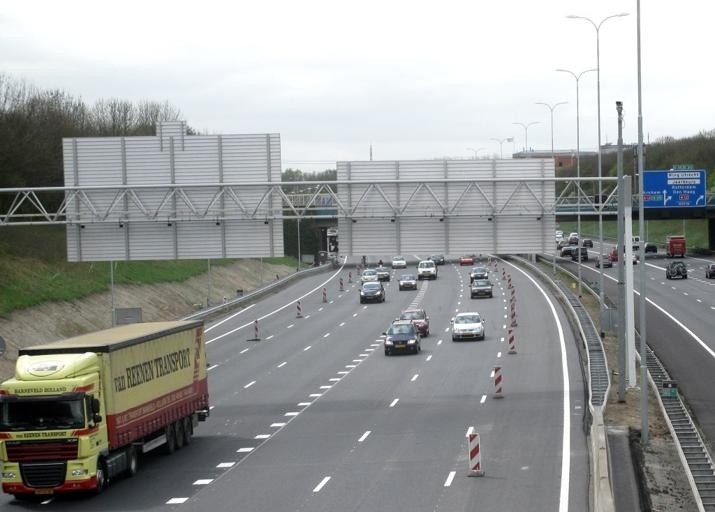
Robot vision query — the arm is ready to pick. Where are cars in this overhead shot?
[357,256,495,363]
[556,229,715,284]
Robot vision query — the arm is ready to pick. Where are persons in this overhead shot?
[331,241,338,252]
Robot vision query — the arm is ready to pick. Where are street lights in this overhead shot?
[469,96,569,160]
[563,10,633,339]
[555,66,602,301]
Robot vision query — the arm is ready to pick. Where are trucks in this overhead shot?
[0,317,212,504]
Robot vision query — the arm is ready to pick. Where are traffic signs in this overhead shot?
[672,163,694,171]
[642,170,707,208]
